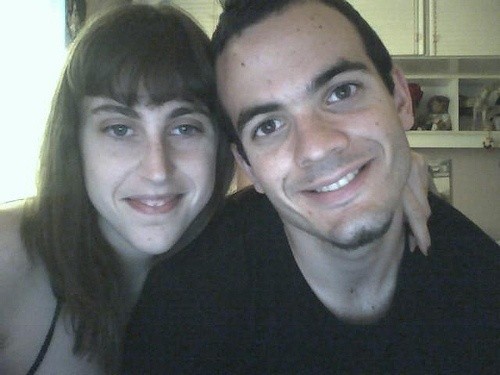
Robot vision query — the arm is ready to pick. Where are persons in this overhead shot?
[0,1,432,374]
[122,0,500,374]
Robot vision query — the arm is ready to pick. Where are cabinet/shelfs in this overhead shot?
[345,0,500,148]
